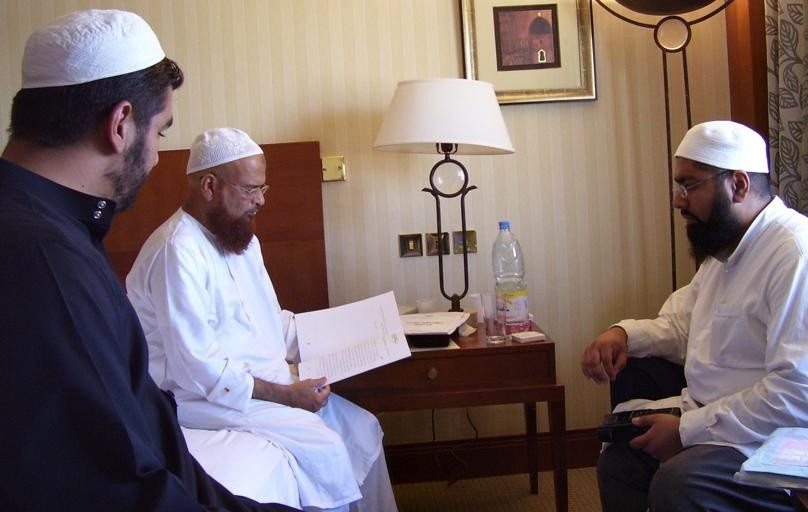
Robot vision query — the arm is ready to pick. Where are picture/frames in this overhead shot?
[459,0,597,104]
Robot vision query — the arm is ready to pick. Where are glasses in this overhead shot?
[673,176,728,194]
[214,173,270,198]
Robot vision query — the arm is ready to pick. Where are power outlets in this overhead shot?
[398,234,422,257]
[453,231,478,254]
[424,232,449,256]
[321,156,345,182]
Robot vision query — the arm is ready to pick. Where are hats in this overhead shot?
[21,8,167,88]
[675,121,771,176]
[185,127,265,174]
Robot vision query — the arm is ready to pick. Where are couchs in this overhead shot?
[611,356,691,417]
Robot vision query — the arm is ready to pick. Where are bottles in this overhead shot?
[491,221,532,334]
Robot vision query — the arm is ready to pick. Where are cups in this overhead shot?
[482,291,507,345]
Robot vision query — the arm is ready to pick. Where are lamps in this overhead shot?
[372,77,518,336]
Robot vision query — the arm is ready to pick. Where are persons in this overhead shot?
[581,120,808,512]
[125,126,399,512]
[0,9,303,512]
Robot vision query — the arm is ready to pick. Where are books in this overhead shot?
[295,290,411,389]
[731,426,808,489]
[511,330,545,343]
[408,337,460,352]
[400,312,471,334]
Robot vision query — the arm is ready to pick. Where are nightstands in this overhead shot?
[331,318,568,512]
